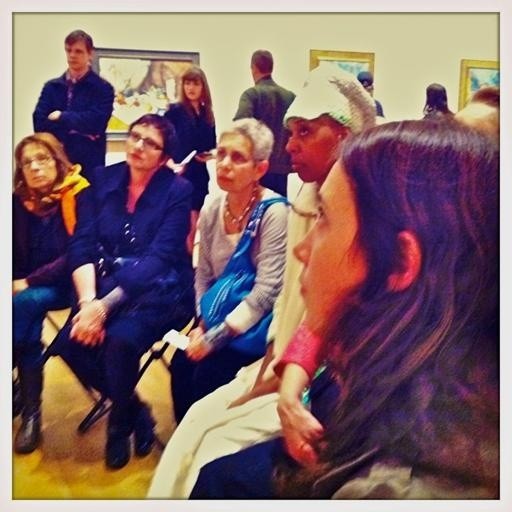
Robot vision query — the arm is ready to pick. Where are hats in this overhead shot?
[283,62,377,136]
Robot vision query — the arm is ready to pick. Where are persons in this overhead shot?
[13,132,88,455]
[189,321,343,500]
[59,115,192,470]
[170,117,287,424]
[274,118,499,498]
[353,69,383,117]
[147,60,376,500]
[420,82,454,125]
[163,66,215,254]
[231,49,295,197]
[33,29,115,170]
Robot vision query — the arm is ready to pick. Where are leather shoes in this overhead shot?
[15,409,41,454]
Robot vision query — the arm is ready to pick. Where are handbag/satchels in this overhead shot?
[113,253,181,294]
[309,363,363,449]
[200,245,274,367]
[94,241,112,277]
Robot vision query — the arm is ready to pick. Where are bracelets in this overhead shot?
[76,294,97,307]
[202,321,235,348]
[94,301,107,323]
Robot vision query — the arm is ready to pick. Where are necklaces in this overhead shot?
[224,184,259,231]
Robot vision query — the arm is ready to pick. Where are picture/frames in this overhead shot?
[458,58,500,111]
[309,49,375,84]
[89,48,199,140]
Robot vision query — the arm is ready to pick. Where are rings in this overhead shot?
[297,439,308,451]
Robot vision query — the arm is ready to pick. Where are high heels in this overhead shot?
[105,424,131,469]
[133,404,157,455]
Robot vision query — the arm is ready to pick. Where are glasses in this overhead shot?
[127,131,162,152]
[19,155,54,169]
